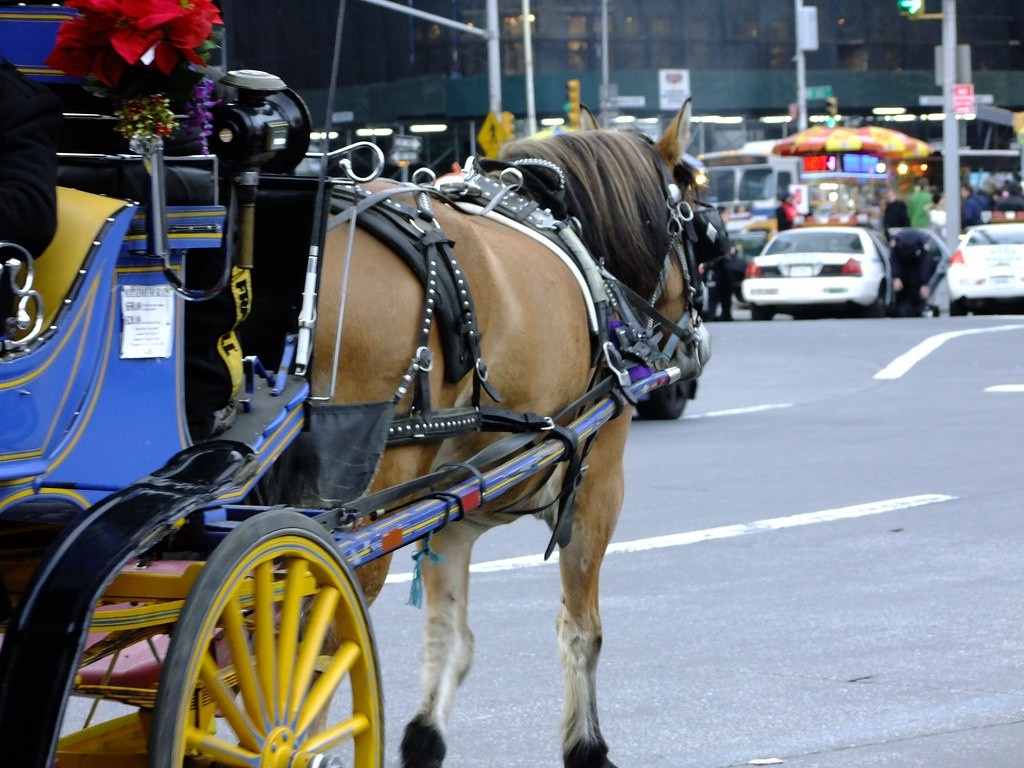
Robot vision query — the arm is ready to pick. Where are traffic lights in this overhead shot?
[899,0,924,16]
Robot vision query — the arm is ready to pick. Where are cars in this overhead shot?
[945,222,1024,316]
[727,226,952,321]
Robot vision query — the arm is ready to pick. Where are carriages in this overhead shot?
[0,1,712,768]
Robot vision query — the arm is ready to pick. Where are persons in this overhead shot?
[960,184,990,229]
[777,191,798,231]
[884,189,912,242]
[0,53,63,339]
[924,192,946,226]
[889,229,934,318]
[977,180,1024,211]
[709,206,737,322]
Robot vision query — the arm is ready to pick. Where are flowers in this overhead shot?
[45,0,224,143]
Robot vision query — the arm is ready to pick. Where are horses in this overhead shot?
[213,96,712,766]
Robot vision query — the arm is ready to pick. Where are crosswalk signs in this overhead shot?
[477,110,511,159]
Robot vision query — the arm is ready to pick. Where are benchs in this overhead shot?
[0,184,141,484]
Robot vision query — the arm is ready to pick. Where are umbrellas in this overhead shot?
[772,122,934,163]
